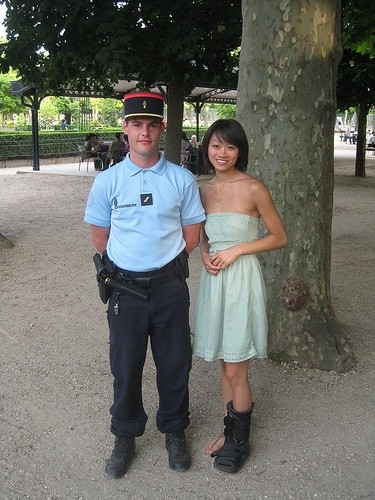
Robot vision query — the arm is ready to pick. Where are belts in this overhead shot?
[117,266,180,288]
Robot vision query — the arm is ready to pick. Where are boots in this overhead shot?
[211,401,254,472]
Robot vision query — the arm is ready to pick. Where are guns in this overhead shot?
[92,252,107,304]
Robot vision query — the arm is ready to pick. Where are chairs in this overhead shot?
[76,144,102,172]
[105,148,124,170]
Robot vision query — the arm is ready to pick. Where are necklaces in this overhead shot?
[215,170,237,206]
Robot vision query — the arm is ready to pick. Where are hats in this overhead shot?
[123,92,165,123]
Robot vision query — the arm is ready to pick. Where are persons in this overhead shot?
[61,120,199,171]
[193,118,289,474]
[338,121,375,155]
[83,92,207,477]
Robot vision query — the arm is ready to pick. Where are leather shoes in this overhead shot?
[105,436,136,479]
[165,432,190,471]
